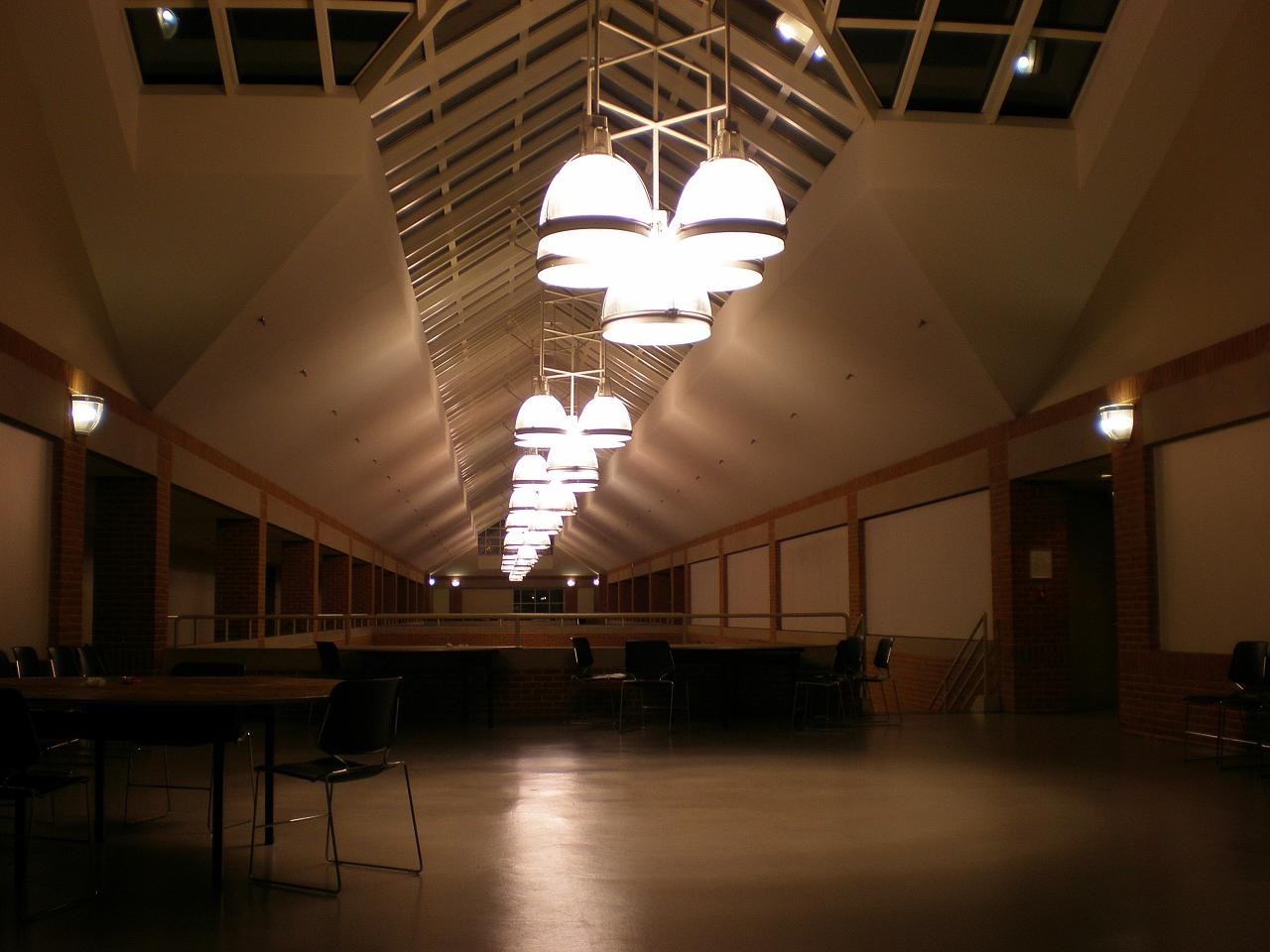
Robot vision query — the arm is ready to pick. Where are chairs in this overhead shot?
[125,660,261,834]
[314,641,345,679]
[0,646,108,913]
[619,638,691,736]
[791,635,902,731]
[563,636,628,725]
[1182,642,1270,779]
[247,677,423,895]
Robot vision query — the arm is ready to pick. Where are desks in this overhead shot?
[0,677,347,893]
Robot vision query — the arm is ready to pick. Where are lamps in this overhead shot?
[71,394,104,434]
[160,7,179,40]
[500,0,786,582]
[1100,403,1135,442]
[775,12,827,57]
